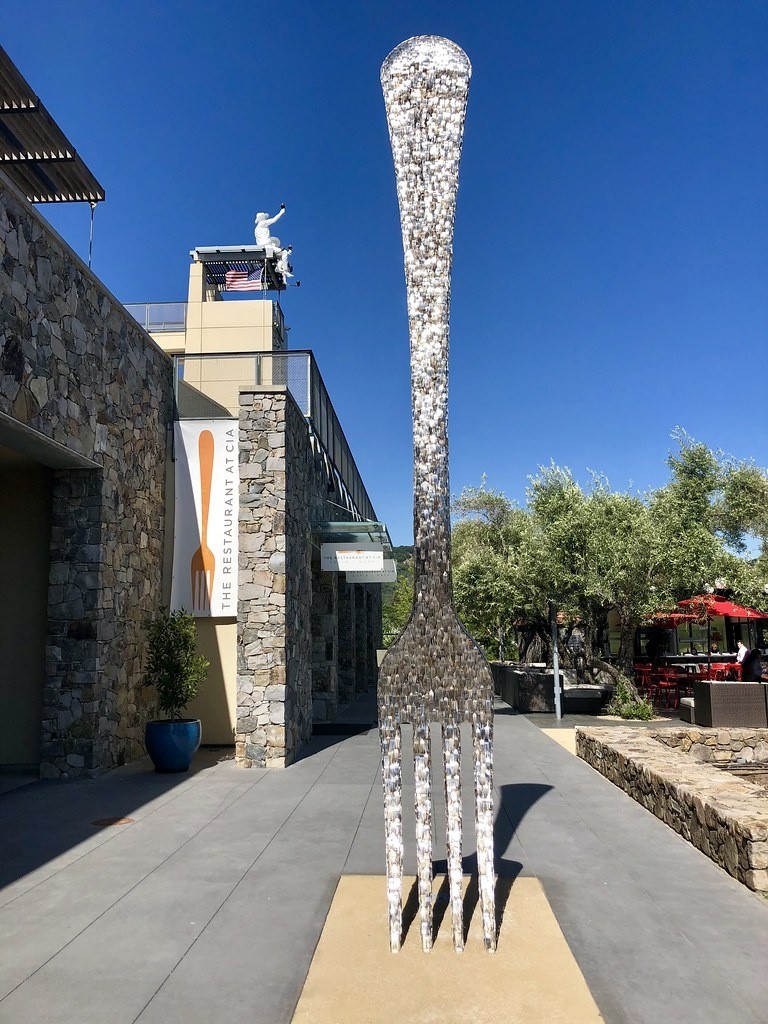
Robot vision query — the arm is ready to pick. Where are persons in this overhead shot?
[736,638,750,663]
[254,208,294,285]
[740,649,763,683]
[710,641,720,652]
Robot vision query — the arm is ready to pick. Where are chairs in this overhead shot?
[634,662,743,709]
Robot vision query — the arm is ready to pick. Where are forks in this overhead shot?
[376,36,501,952]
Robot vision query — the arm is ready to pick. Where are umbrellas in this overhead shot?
[678,593,733,673]
[706,595,768,650]
[642,610,702,655]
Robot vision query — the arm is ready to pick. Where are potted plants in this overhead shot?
[141,605,211,775]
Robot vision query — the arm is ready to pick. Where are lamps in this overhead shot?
[274,244,301,288]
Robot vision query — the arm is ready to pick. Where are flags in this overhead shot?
[226,268,264,291]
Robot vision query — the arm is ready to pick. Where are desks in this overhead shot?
[650,673,694,686]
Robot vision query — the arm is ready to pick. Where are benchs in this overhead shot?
[680,680,768,728]
